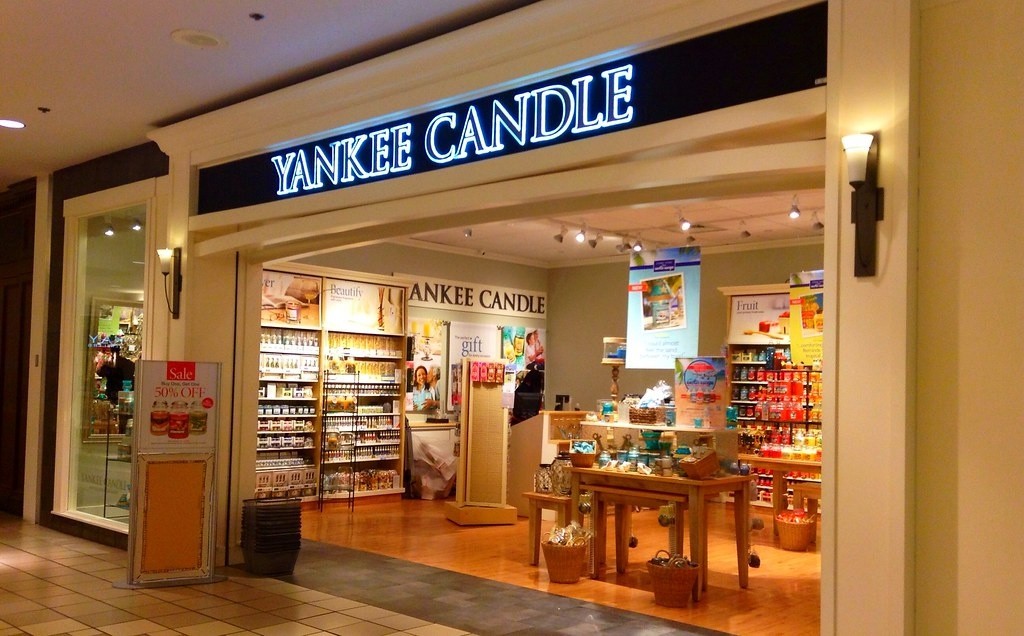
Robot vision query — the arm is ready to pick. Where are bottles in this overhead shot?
[675,556,688,568]
[326,383,401,394]
[737,425,823,462]
[323,415,400,462]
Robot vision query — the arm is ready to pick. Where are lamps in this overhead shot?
[788,195,801,219]
[841,134,885,277]
[633,232,643,251]
[157,248,182,320]
[553,225,568,242]
[588,232,603,248]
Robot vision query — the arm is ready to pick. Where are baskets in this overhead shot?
[569,453,595,468]
[645,550,701,608]
[679,450,721,480]
[240,496,302,576]
[541,532,589,583]
[774,505,816,551]
[628,406,666,425]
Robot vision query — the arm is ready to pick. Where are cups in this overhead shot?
[694,419,702,428]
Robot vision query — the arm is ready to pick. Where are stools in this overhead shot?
[522,491,572,567]
[786,482,822,515]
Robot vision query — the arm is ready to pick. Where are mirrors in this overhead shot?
[82,294,143,444]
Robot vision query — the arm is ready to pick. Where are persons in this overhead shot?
[524,329,545,370]
[96,345,135,434]
[413,366,440,411]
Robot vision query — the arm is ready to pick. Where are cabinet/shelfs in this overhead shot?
[727,343,822,517]
[325,328,405,509]
[257,324,324,511]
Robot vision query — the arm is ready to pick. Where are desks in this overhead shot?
[562,464,758,601]
[738,453,822,546]
[580,421,727,461]
